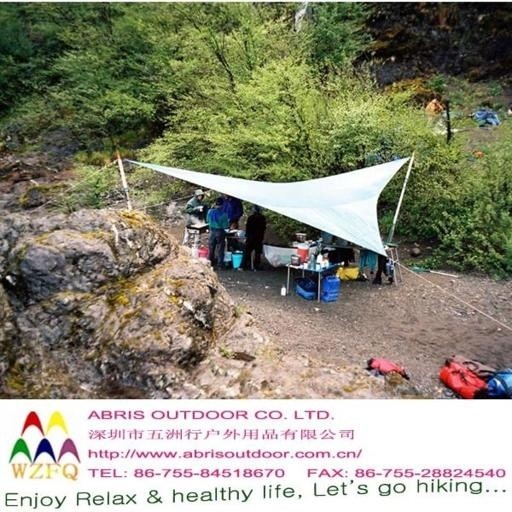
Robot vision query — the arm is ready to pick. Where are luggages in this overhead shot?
[293,277,318,300]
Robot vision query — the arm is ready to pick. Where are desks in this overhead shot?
[285,261,338,303]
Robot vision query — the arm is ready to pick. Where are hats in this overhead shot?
[195,189,204,197]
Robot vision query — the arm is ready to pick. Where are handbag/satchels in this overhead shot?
[480,368,512,399]
[337,266,359,280]
[368,357,409,379]
[441,358,488,399]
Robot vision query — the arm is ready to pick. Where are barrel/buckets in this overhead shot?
[197,246,208,256]
[321,275,341,302]
[297,243,310,263]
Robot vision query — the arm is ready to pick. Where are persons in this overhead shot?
[425,95,443,120]
[185,189,266,271]
